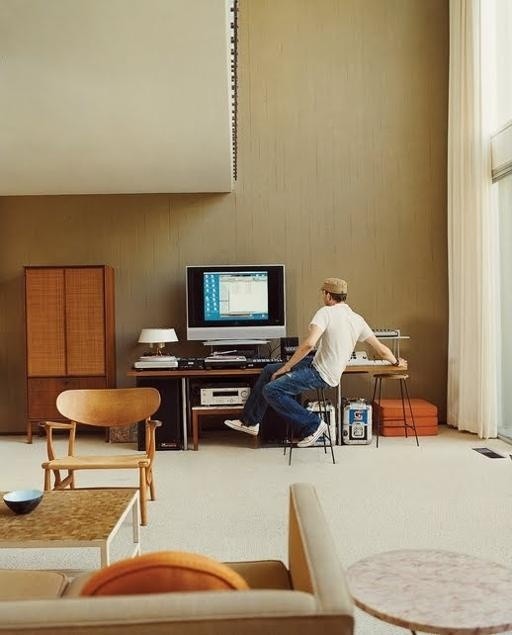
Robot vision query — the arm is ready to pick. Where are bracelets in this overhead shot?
[393,360,401,368]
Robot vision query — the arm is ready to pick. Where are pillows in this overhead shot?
[79,551,250,597]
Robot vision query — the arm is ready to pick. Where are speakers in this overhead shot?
[136,377,183,451]
[280,337,299,359]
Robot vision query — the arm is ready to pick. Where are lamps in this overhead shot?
[137,327,179,360]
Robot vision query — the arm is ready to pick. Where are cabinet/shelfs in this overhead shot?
[23,264,117,444]
[191,405,266,451]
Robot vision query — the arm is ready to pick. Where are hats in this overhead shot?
[321,278,347,294]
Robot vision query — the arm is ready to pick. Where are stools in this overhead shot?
[283,387,335,464]
[370,373,419,448]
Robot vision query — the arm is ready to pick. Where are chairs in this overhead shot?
[39,386,162,526]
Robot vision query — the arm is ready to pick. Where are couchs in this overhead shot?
[0,482,355,635]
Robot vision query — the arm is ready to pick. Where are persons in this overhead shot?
[224,278,406,447]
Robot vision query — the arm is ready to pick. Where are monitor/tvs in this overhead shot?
[186,264,287,346]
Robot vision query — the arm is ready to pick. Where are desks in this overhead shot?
[345,549,512,635]
[125,357,409,450]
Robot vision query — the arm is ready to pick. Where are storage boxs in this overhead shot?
[342,395,373,445]
[303,398,336,447]
[374,397,438,436]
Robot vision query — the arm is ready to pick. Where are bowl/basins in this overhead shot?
[4,489,44,515]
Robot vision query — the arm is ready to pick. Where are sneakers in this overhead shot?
[298,420,327,447]
[224,420,260,435]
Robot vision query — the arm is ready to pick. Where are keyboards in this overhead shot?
[243,358,283,368]
